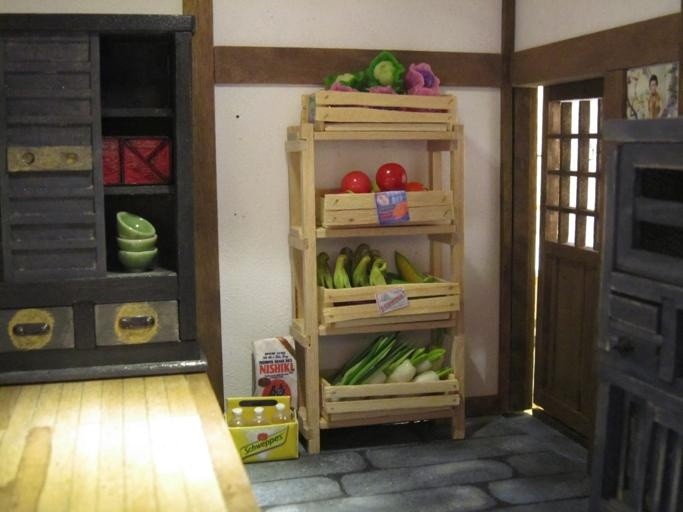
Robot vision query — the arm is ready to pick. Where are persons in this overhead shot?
[645,73,665,119]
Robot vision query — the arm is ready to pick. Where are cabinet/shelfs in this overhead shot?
[585,117,682,512]
[1,10,210,388]
[284,121,468,456]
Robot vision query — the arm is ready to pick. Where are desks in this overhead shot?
[1,370,260,511]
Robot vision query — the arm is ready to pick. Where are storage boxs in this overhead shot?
[102,134,170,188]
[222,406,300,464]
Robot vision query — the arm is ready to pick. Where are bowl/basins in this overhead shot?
[116,211,159,271]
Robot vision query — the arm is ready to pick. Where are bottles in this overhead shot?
[229,402,291,427]
